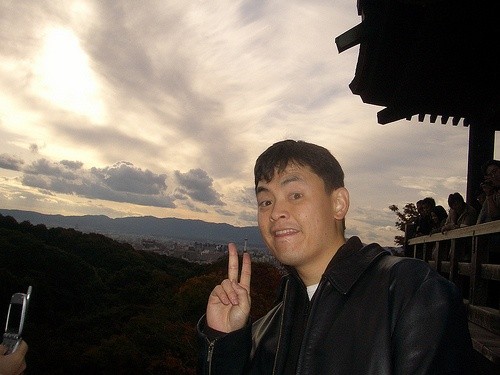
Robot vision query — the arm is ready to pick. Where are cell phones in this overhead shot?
[2,285,32,356]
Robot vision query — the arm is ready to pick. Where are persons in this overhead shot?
[193,139,473,375]
[415,160,500,265]
[0,340,27,375]
